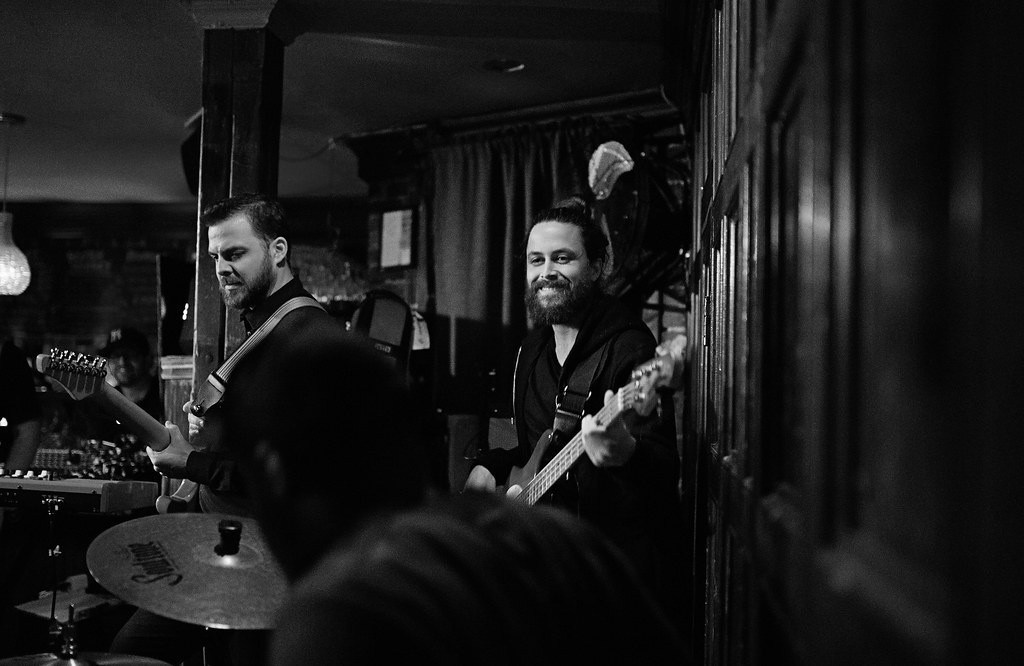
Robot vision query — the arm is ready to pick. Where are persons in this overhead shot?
[110,190,413,666]
[271,491,691,666]
[96,327,165,427]
[462,207,683,638]
[0,371,41,661]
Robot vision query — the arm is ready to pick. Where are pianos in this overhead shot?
[0,464,158,511]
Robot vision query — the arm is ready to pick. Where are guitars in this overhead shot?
[29,347,202,514]
[486,354,681,507]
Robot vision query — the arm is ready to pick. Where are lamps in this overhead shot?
[0,113,32,296]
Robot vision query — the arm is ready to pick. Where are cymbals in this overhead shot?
[1,652,175,666]
[85,510,299,633]
